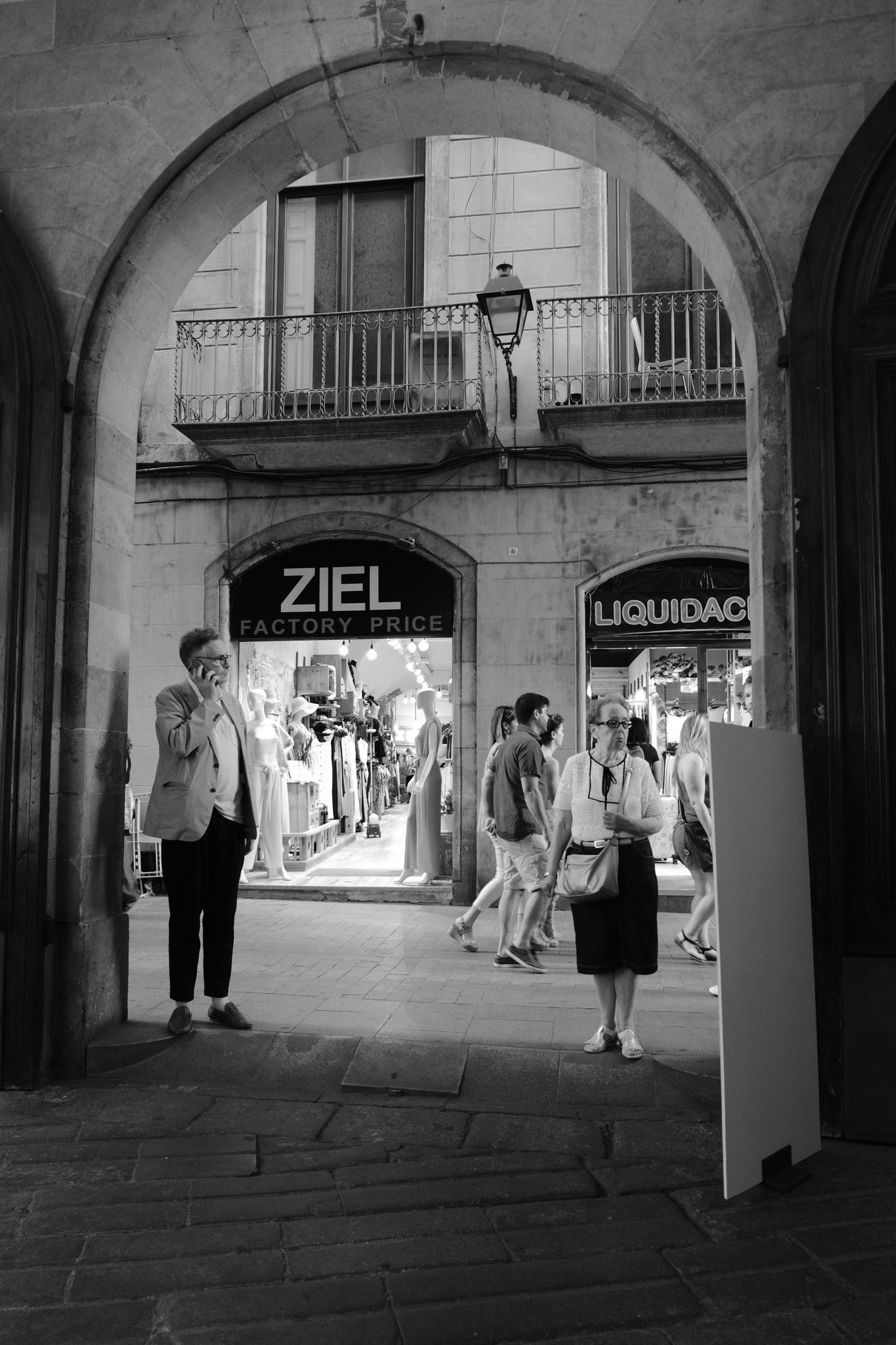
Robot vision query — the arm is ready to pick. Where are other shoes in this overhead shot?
[709,985,718,996]
[531,929,560,947]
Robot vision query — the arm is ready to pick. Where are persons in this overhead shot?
[671,712,717,963]
[239,682,447,888]
[449,693,564,974]
[539,690,665,1060]
[741,675,752,727]
[625,716,662,792]
[143,626,260,1037]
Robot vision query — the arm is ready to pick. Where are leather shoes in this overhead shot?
[168,1004,197,1036]
[207,1001,253,1030]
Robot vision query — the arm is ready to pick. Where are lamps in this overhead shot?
[394,537,417,552]
[387,638,431,688]
[259,542,276,556]
[476,262,534,419]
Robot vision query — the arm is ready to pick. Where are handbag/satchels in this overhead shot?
[667,741,679,755]
[553,835,619,903]
[683,819,713,873]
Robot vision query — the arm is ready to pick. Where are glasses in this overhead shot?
[194,655,231,666]
[595,720,633,729]
[647,652,733,706]
[551,714,557,726]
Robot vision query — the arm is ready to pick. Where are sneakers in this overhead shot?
[505,942,548,973]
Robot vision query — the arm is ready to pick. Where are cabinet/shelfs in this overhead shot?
[286,779,319,845]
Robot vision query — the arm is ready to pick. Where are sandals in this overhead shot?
[674,930,706,963]
[493,953,525,968]
[449,916,478,952]
[584,1023,619,1053]
[530,938,550,950]
[616,1021,643,1059]
[695,940,717,961]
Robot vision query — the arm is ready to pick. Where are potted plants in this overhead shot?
[285,731,323,778]
[441,790,453,832]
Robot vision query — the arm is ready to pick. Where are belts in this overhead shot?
[571,835,647,848]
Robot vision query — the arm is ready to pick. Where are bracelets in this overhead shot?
[544,872,557,878]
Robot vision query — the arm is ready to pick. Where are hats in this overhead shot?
[290,696,320,721]
[263,689,280,703]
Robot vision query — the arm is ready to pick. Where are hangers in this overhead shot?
[441,759,452,770]
[660,698,695,717]
[446,721,452,738]
[628,705,648,717]
[707,698,726,710]
[353,757,389,772]
[306,715,369,737]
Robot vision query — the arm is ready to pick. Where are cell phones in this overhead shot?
[188,660,219,687]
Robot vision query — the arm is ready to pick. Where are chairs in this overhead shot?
[631,317,695,398]
